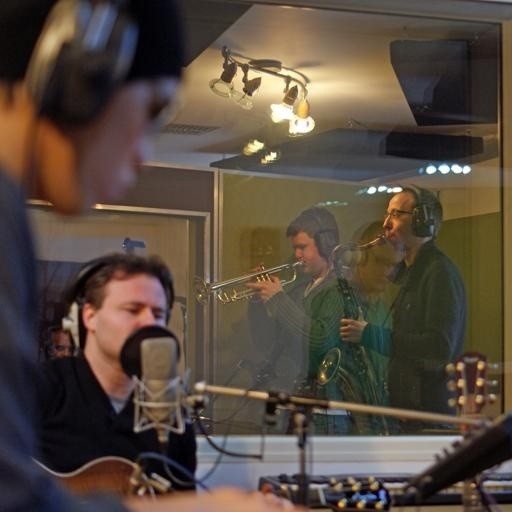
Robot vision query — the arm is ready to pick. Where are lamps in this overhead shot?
[289,90,316,136]
[269,76,299,123]
[228,65,262,110]
[210,49,238,98]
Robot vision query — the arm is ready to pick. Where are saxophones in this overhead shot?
[315,236,389,437]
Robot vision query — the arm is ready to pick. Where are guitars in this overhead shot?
[445,352,499,512]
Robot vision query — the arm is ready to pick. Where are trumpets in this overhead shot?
[191,259,305,306]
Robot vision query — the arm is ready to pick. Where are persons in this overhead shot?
[245,206,393,435]
[46,330,74,362]
[35,251,199,491]
[339,184,466,434]
[1,0,302,511]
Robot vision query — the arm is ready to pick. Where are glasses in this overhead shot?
[385,207,412,219]
[51,341,71,353]
[143,79,180,137]
